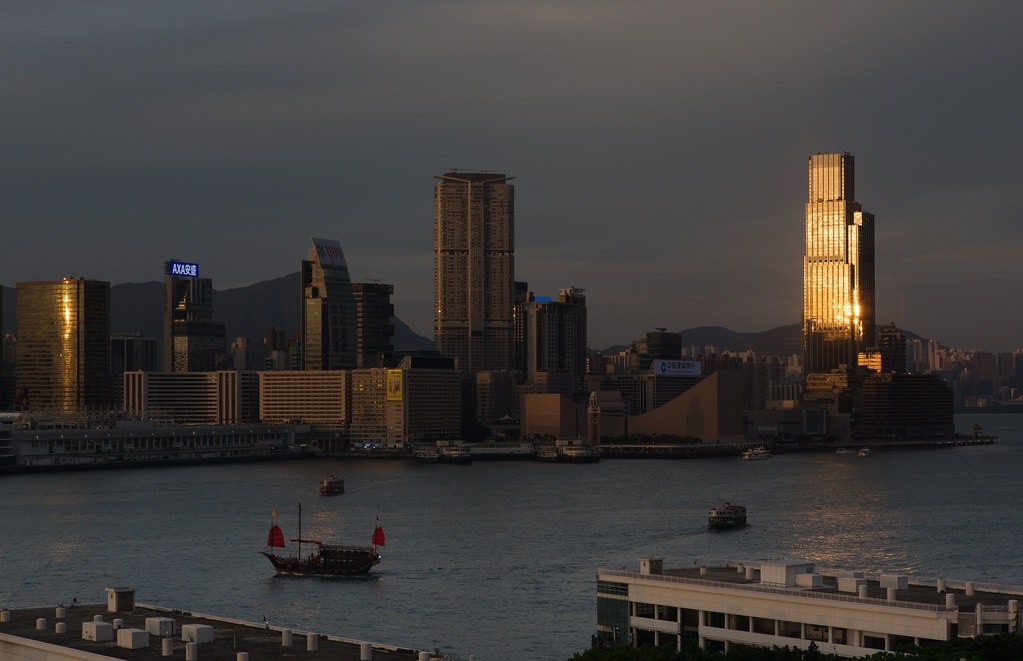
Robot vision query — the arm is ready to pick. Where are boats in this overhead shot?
[417,453,440,463]
[707,502,747,531]
[319,474,345,497]
[741,445,771,460]
[858,447,873,457]
[259,500,386,576]
[443,445,474,465]
[834,447,857,455]
